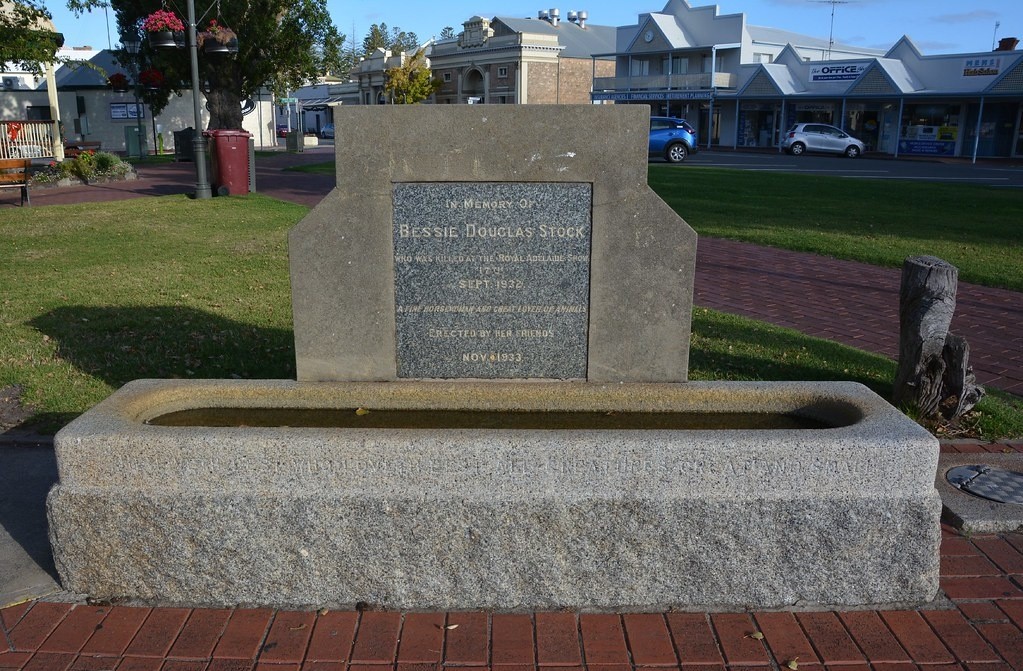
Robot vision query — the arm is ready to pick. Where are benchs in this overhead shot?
[0,140,101,158]
[0,158,32,206]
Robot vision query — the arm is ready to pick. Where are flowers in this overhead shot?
[195,17,237,49]
[138,10,187,33]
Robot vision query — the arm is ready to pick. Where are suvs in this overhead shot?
[648,116,699,164]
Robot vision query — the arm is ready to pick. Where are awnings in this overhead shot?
[304,97,343,112]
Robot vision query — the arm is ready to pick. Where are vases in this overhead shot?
[204,38,240,53]
[148,29,185,50]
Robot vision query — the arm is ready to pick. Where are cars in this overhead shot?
[321,122,334,139]
[276,124,294,138]
[781,122,866,159]
[9,132,83,159]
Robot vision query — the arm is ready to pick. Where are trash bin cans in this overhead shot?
[173,127,196,156]
[202,129,255,196]
